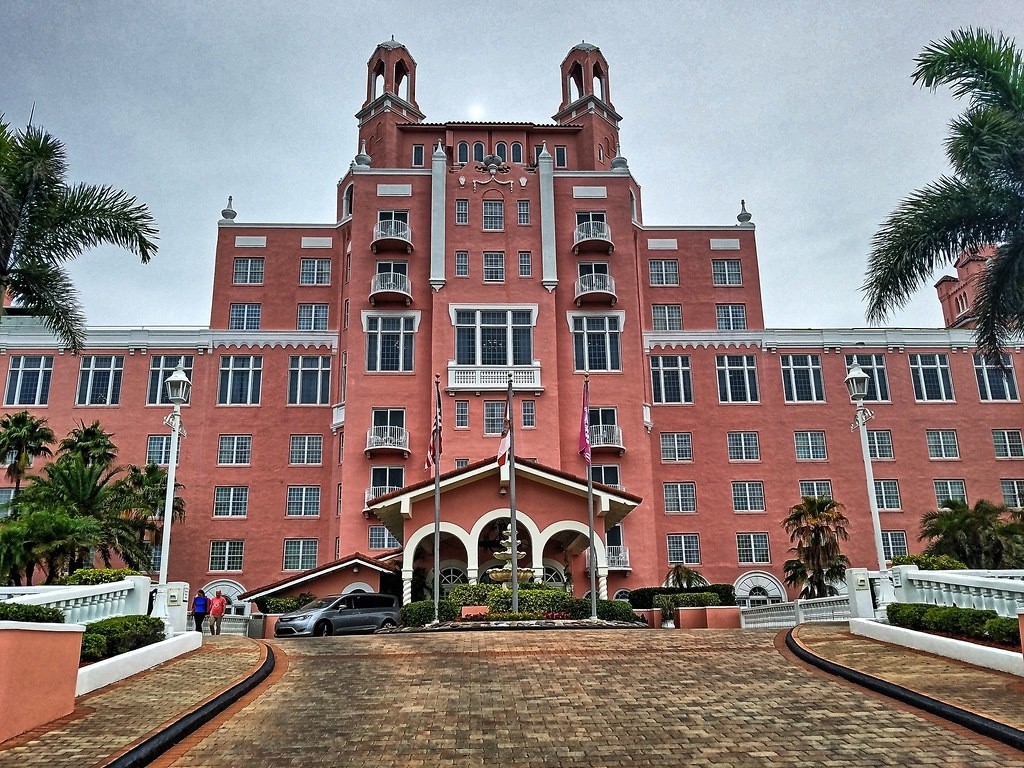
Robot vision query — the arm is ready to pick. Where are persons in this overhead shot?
[206,590,226,635]
[190,590,211,632]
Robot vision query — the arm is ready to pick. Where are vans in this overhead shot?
[274,594,399,637]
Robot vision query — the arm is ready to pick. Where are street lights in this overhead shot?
[149,362,194,638]
[843,354,899,623]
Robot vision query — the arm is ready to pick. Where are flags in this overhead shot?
[497,394,511,487]
[577,382,591,468]
[425,387,443,471]
